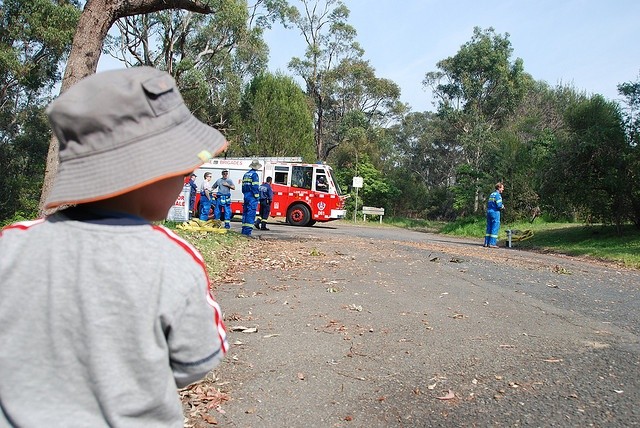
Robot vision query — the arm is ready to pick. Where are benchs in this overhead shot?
[362,206,385,224]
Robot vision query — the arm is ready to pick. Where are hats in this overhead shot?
[249,159,262,170]
[44,66,229,210]
[222,169,228,172]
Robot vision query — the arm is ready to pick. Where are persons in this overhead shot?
[241,159,260,236]
[316,177,329,190]
[199,172,213,221]
[211,170,235,229]
[483,182,505,248]
[0,66,229,428]
[188,174,197,220]
[254,177,273,231]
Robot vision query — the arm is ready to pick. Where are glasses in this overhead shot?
[222,172,228,175]
[206,176,211,178]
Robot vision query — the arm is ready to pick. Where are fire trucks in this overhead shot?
[191,156,347,226]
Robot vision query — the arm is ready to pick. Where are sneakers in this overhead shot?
[225,223,230,228]
[262,225,269,230]
[254,222,260,229]
